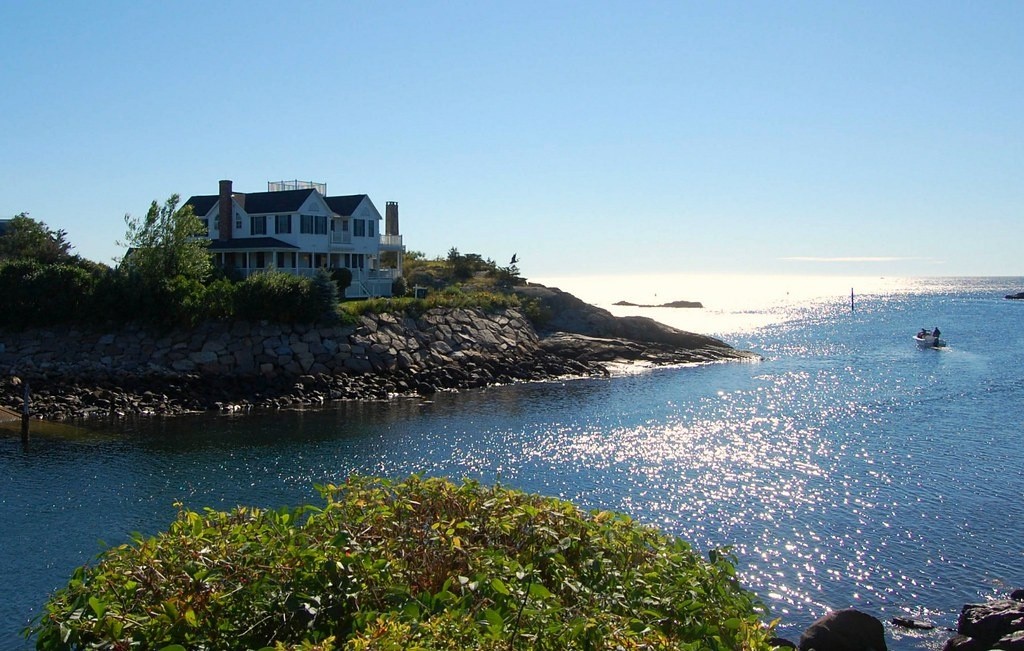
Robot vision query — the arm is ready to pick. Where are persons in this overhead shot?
[933,326,941,342]
[919,327,929,338]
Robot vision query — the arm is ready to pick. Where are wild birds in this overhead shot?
[509,253,520,264]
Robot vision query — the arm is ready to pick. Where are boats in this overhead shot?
[912,328,946,350]
[1004,292,1024,299]
